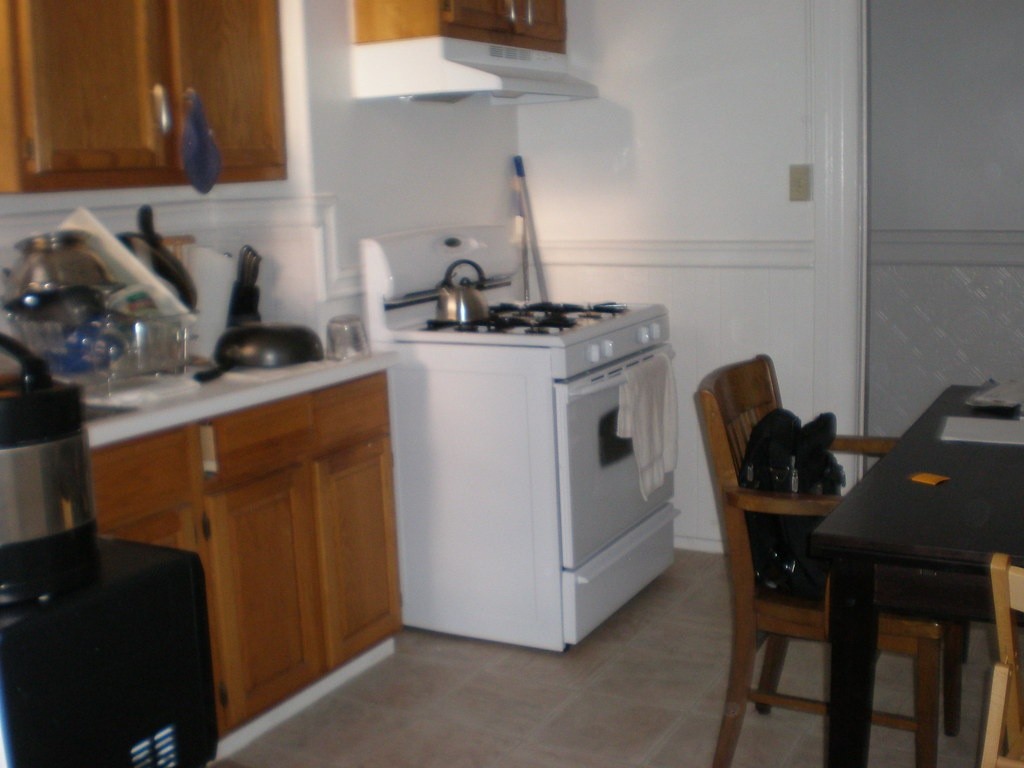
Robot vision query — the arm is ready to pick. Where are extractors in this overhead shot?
[348,36,600,108]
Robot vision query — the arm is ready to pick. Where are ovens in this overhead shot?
[388,306,680,653]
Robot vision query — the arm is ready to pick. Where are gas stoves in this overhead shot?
[362,222,669,347]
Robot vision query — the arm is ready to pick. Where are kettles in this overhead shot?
[436,259,489,322]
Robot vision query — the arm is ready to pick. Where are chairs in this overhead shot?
[698,354,969,768]
[982,553,1024,768]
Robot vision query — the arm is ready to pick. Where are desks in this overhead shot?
[815,385,1024,768]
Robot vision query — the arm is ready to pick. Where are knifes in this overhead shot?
[236,244,263,292]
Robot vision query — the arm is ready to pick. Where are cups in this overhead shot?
[326,317,370,360]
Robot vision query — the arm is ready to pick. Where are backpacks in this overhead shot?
[738,408,846,600]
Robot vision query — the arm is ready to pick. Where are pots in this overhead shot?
[114,205,196,312]
[193,325,324,384]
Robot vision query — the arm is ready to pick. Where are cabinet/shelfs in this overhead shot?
[88,370,401,737]
[352,1,568,54]
[1,0,288,193]
